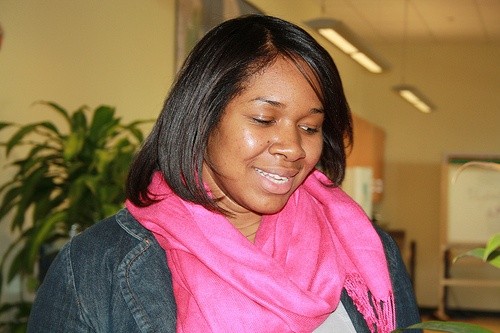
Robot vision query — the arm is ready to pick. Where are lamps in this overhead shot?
[390,0,437,116]
[302,0,394,78]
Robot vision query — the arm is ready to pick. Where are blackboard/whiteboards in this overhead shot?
[441,153,500,243]
